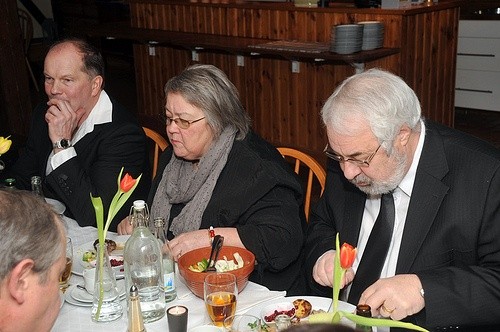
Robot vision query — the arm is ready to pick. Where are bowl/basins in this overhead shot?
[177,243,255,301]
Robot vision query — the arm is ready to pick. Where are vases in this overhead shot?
[90,243,124,322]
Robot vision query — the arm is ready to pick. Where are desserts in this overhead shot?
[293,299,312,318]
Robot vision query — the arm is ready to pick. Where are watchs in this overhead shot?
[52,138,72,149]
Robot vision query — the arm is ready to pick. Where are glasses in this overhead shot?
[322,129,403,169]
[161,116,206,130]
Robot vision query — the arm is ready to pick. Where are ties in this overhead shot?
[347,191,396,307]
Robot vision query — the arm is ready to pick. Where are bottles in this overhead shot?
[355,305,373,332]
[5,178,16,192]
[126,286,146,332]
[31,176,43,197]
[152,217,176,303]
[124,200,167,322]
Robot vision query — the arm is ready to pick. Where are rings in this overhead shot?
[381,305,391,315]
[177,253,181,259]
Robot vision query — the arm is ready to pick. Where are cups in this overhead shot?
[58,237,73,293]
[222,315,261,332]
[83,268,101,294]
[204,273,238,327]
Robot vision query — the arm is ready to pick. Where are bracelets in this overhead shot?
[207,225,215,247]
[420,288,424,299]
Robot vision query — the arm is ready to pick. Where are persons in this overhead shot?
[0,188,68,332]
[306,67,500,331]
[115,64,307,296]
[12,38,148,234]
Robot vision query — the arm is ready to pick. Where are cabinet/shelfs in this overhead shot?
[127,0,462,185]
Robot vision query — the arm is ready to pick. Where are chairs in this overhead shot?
[141,126,169,185]
[277,147,328,224]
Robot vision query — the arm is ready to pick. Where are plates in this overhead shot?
[70,235,131,279]
[238,295,378,332]
[328,20,385,54]
[46,198,66,215]
[59,282,126,309]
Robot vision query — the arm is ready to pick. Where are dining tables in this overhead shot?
[50,215,284,332]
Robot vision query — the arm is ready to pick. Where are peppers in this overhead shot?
[189,258,208,272]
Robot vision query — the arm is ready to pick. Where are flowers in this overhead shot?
[330,233,428,332]
[0,135,12,157]
[91,166,143,321]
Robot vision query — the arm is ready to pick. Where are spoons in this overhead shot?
[206,237,224,272]
